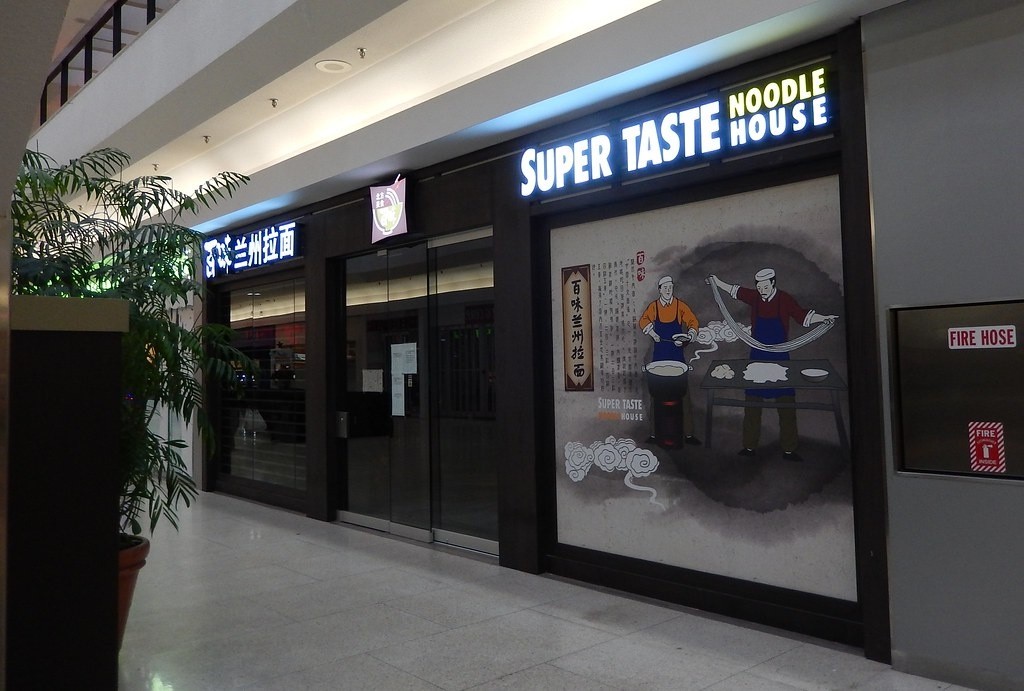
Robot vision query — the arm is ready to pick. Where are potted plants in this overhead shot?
[11,144,257,652]
